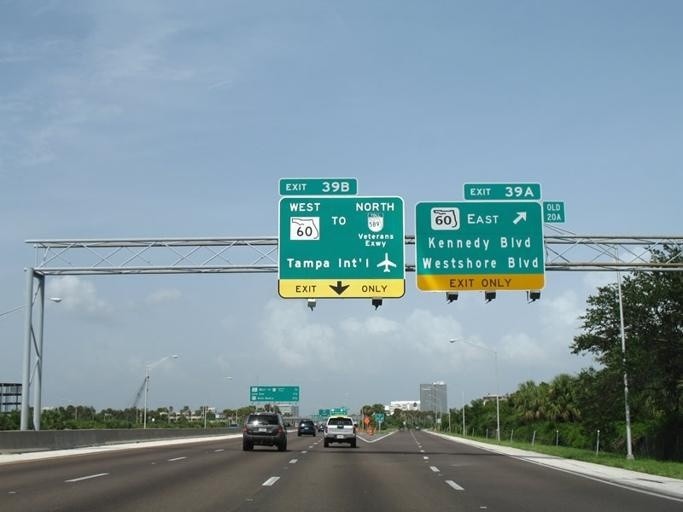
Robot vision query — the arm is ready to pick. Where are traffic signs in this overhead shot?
[317,408,330,416]
[374,413,384,422]
[330,408,347,415]
[248,385,300,402]
[272,171,571,294]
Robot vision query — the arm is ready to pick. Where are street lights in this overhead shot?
[0,295,64,317]
[142,353,180,429]
[203,376,233,428]
[446,338,501,443]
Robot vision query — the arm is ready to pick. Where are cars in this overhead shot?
[414,425,420,430]
[228,422,240,429]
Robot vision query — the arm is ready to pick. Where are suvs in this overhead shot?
[296,415,357,448]
[241,410,289,452]
